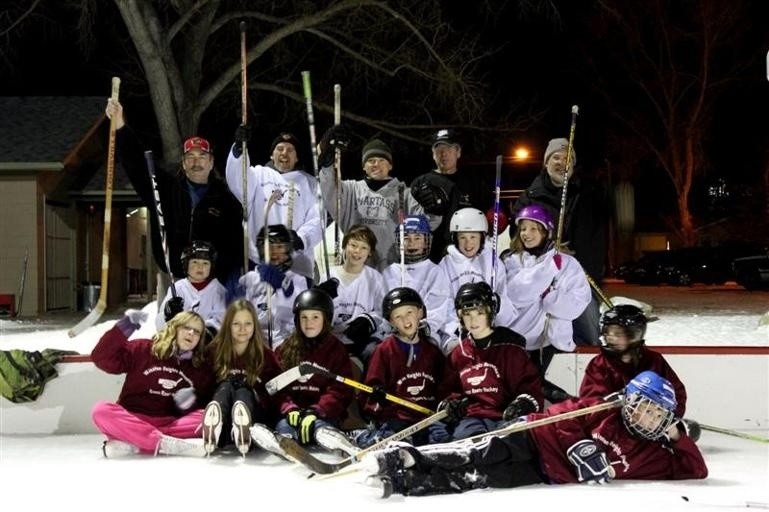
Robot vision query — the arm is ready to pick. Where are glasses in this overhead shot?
[181,324,202,336]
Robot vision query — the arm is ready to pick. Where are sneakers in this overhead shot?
[103,440,140,458]
[367,470,408,500]
[230,401,253,454]
[202,401,223,454]
[361,447,405,478]
[153,435,208,458]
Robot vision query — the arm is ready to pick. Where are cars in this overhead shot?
[613,240,769,289]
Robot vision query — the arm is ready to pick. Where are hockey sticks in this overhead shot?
[302,71,360,334]
[525,106,579,350]
[279,410,448,474]
[68,76,120,338]
[262,189,284,351]
[264,365,434,418]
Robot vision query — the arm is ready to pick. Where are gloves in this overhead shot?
[124,308,147,326]
[297,409,318,447]
[553,254,561,270]
[235,123,440,216]
[164,297,184,322]
[567,439,616,485]
[446,398,468,420]
[204,326,217,346]
[368,384,386,405]
[503,398,537,422]
[257,264,374,343]
[173,388,196,410]
[285,407,302,428]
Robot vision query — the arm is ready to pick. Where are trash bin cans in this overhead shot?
[82,285,100,313]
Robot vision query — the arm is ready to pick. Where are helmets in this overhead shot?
[256,224,293,273]
[514,204,555,231]
[180,239,218,277]
[393,214,434,264]
[621,370,677,442]
[598,305,647,355]
[382,287,424,321]
[449,207,489,233]
[454,282,493,309]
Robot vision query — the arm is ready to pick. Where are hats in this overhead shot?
[183,136,210,153]
[431,128,459,149]
[544,137,577,166]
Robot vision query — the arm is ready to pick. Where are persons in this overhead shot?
[364,286,453,447]
[105,98,602,343]
[348,368,709,500]
[577,294,687,419]
[269,285,356,460]
[440,280,547,443]
[89,308,221,458]
[192,297,275,458]
